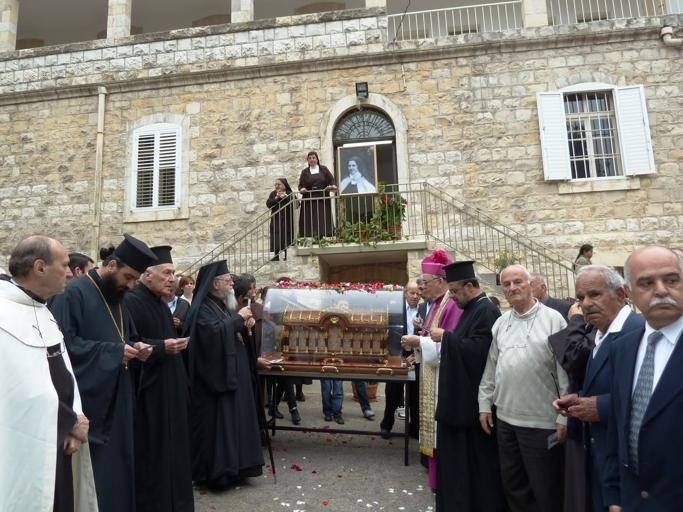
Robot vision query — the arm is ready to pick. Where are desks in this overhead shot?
[257,357,417,468]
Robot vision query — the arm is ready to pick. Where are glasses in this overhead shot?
[423,278,440,286]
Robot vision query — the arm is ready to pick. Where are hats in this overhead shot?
[422,249,452,276]
[145,243,174,267]
[114,233,158,275]
[199,260,231,278]
[441,260,477,284]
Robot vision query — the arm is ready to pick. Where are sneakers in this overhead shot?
[395,407,406,420]
[290,408,301,423]
[381,427,392,439]
[364,410,375,418]
[268,409,284,419]
[324,414,333,422]
[333,415,345,424]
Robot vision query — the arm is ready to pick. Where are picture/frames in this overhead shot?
[337,144,379,197]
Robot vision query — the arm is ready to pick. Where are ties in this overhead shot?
[627,331,663,477]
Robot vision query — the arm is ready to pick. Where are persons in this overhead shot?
[229,274,249,309]
[267,376,312,424]
[412,279,434,335]
[552,265,646,512]
[280,376,313,402]
[352,379,376,418]
[319,380,346,424]
[0,235,101,512]
[180,276,196,305]
[67,252,94,278]
[276,276,291,284]
[124,245,191,512]
[378,281,419,439]
[547,275,625,384]
[397,382,409,419]
[266,177,300,261]
[607,246,683,512]
[260,286,275,304]
[572,244,593,274]
[336,300,349,311]
[477,265,572,512]
[166,276,190,333]
[299,152,337,237]
[527,273,574,324]
[188,260,265,493]
[339,157,377,193]
[489,296,500,309]
[429,259,503,512]
[47,232,153,512]
[668,248,683,257]
[400,249,464,496]
[241,273,263,352]
